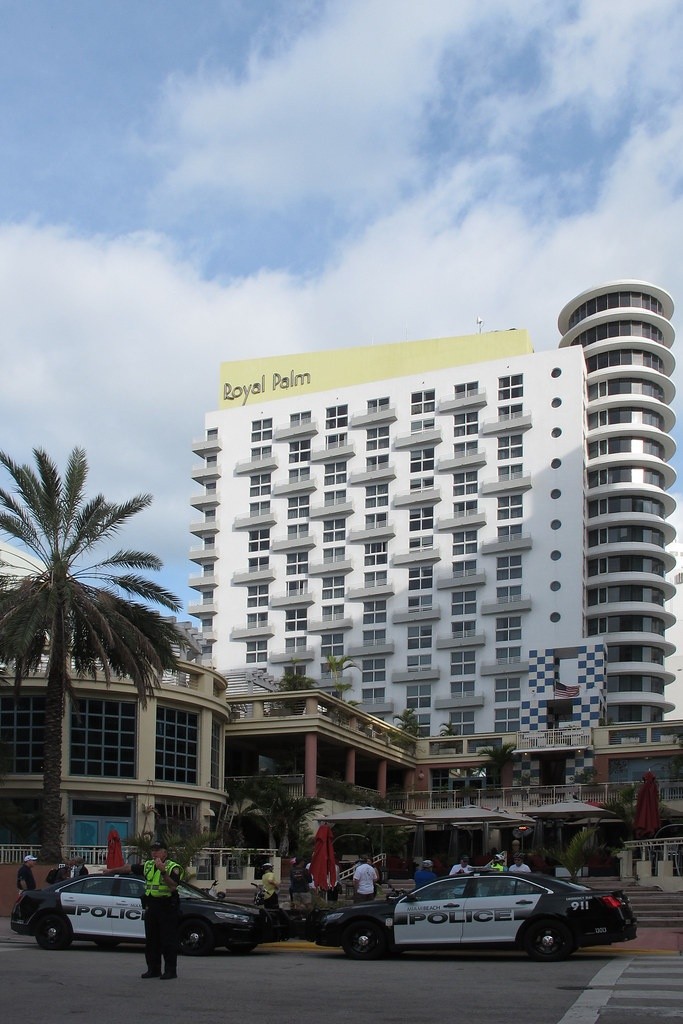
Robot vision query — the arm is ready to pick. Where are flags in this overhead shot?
[555,681,579,698]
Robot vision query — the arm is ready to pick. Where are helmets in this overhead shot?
[514,852,526,859]
[422,860,433,868]
[493,854,506,863]
[263,863,274,868]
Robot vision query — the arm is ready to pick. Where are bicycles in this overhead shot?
[250,882,271,907]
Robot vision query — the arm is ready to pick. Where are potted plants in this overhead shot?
[621,733,640,744]
[660,730,679,743]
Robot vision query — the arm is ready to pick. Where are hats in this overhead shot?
[24,855,37,862]
[150,841,167,849]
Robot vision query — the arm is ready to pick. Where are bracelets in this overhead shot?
[161,871,166,875]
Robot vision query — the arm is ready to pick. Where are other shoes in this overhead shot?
[142,969,162,978]
[160,969,177,980]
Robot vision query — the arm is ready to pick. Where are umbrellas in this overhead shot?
[309,822,336,902]
[314,798,616,865]
[633,771,660,839]
[107,829,124,867]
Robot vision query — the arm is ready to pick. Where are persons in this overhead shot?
[509,853,532,892]
[327,865,340,901]
[449,855,473,897]
[98,841,185,980]
[16,855,37,893]
[55,857,88,882]
[353,855,379,904]
[488,854,509,890]
[262,863,279,908]
[289,857,321,906]
[414,859,437,888]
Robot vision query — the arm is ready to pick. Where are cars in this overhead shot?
[10,872,272,957]
[314,865,638,961]
